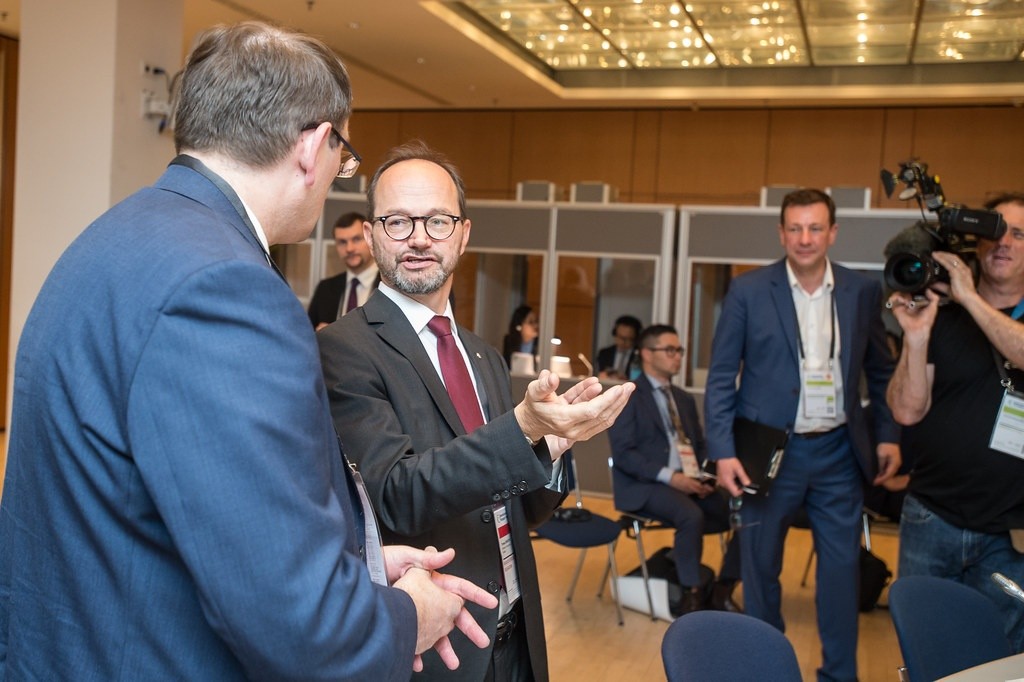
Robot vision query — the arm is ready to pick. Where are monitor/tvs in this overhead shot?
[509,353,571,379]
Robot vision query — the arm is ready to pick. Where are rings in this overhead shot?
[954,261,959,268]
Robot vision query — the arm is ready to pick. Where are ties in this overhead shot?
[427,315,485,434]
[346,279,361,314]
[658,387,687,445]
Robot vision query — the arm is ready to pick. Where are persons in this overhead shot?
[704,188,902,682]
[317,140,638,682]
[0,20,500,682]
[502,304,542,371]
[308,211,381,334]
[597,315,644,382]
[609,324,745,615]
[883,192,1024,653]
[863,331,902,401]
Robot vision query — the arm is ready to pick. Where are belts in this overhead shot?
[793,423,846,439]
[496,599,522,644]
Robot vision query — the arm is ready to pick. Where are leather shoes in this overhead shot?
[684,587,742,613]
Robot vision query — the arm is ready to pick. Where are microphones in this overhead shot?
[882,226,931,262]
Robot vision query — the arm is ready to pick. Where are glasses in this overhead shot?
[372,213,463,240]
[302,123,361,178]
[649,345,684,358]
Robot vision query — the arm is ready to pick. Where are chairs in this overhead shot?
[530,461,626,625]
[597,458,730,622]
